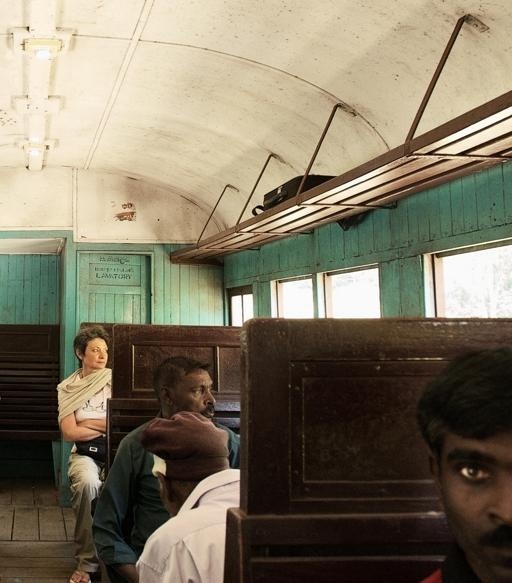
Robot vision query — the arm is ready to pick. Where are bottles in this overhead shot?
[139,410,231,479]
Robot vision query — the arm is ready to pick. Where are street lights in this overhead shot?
[74,436,108,459]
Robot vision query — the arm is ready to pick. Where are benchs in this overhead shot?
[222,318,510,581]
[79,318,244,482]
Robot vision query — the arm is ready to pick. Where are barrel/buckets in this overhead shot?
[71,570,92,582]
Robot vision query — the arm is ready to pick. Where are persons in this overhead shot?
[416,340,510,581]
[133,410,242,583]
[91,355,240,581]
[55,323,115,582]
